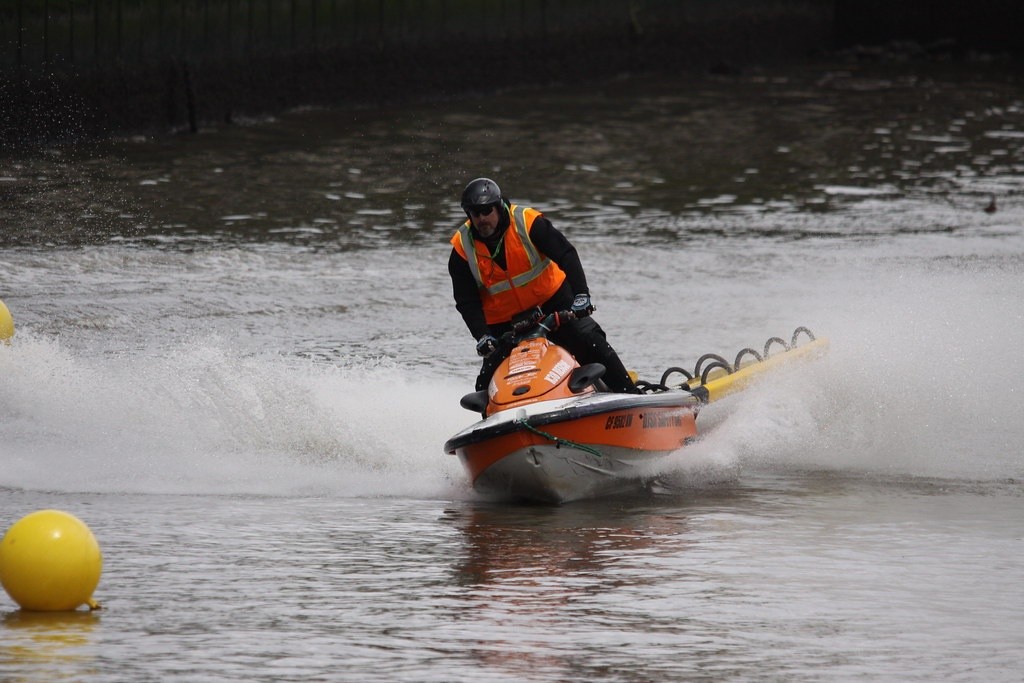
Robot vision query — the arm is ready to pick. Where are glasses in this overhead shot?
[469,203,497,217]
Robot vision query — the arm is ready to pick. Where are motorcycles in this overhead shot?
[443,297,851,516]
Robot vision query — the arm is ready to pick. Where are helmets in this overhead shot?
[460,178,502,213]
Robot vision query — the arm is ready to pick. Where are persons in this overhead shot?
[449,178,646,420]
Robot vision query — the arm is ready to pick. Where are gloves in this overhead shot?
[569,293,593,319]
[476,334,498,358]
